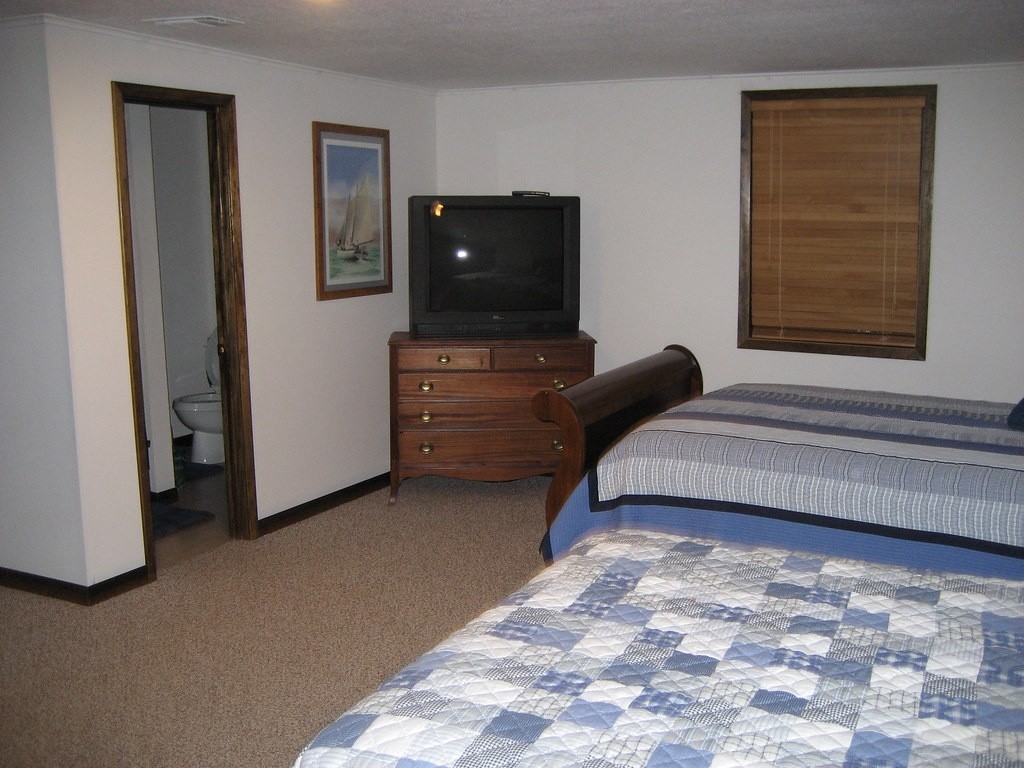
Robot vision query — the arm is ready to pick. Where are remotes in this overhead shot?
[511,191,550,196]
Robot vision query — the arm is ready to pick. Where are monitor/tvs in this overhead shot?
[407,195,580,337]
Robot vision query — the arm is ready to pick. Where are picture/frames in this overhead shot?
[311,121,394,301]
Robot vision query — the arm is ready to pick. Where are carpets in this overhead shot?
[151,502,217,543]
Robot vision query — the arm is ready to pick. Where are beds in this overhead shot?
[292,528,1024,767]
[530,342,1024,580]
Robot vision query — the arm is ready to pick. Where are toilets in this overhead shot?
[172,327,225,464]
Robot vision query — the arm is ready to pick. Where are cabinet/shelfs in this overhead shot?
[387,331,598,506]
